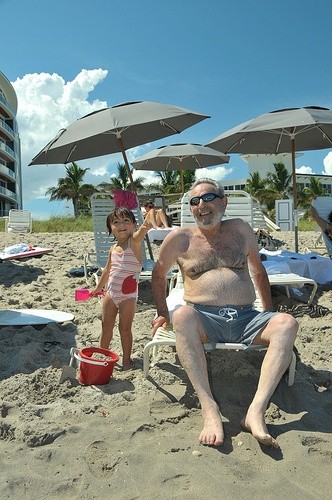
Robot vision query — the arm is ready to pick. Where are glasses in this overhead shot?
[147,205,154,208]
[190,193,223,206]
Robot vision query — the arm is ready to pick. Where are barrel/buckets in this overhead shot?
[73,346,119,386]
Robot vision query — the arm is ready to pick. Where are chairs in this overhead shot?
[83,190,318,386]
[4,209,33,245]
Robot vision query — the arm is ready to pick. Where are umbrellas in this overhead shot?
[130,143,231,197]
[28,100,211,260]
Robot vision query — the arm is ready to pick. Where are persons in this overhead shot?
[204,105,332,253]
[310,195,332,257]
[143,201,169,229]
[90,207,153,371]
[150,177,299,449]
